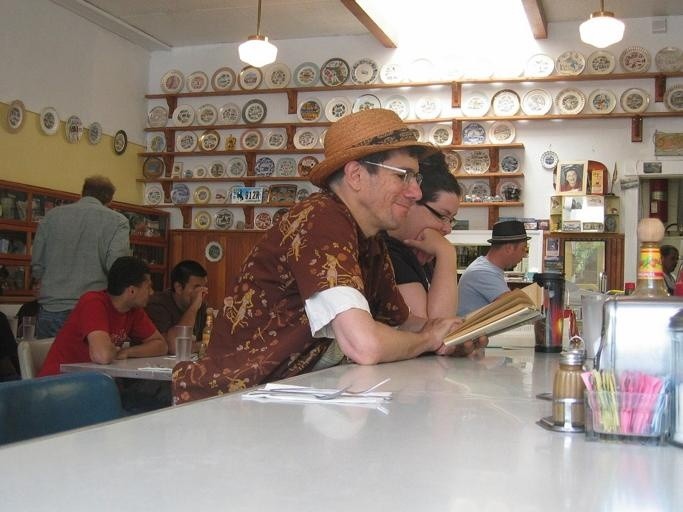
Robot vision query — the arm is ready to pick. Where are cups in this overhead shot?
[175,325,193,363]
[22,316,36,340]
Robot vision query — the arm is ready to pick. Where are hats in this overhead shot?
[308,107,434,189]
[487,220,532,244]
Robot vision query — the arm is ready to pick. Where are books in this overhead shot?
[443,283,547,346]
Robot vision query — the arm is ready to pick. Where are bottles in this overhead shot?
[533,273,564,353]
[553,349,585,428]
[626,281,634,295]
[201,307,220,345]
[197,342,206,358]
[630,241,670,297]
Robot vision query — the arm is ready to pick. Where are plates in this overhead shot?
[276,157,298,176]
[663,84,683,112]
[226,156,247,177]
[588,88,616,113]
[218,102,241,125]
[500,153,520,173]
[193,186,212,205]
[324,96,351,123]
[196,104,218,125]
[186,71,210,92]
[462,120,489,144]
[293,61,320,86]
[214,209,234,230]
[555,49,585,76]
[496,178,521,202]
[212,189,229,205]
[194,210,212,230]
[655,47,683,71]
[172,105,195,126]
[237,65,263,91]
[39,107,60,136]
[414,92,441,120]
[176,131,198,152]
[205,240,224,262]
[540,151,559,169]
[254,211,272,231]
[254,157,275,176]
[320,57,350,86]
[521,87,553,115]
[491,89,521,116]
[429,125,453,146]
[382,94,410,120]
[114,129,128,155]
[489,120,516,144]
[7,100,27,133]
[467,181,492,201]
[211,67,236,91]
[461,89,491,118]
[443,151,462,174]
[457,182,466,201]
[161,70,185,93]
[147,106,168,128]
[586,50,616,74]
[193,165,207,178]
[380,62,405,84]
[265,63,291,88]
[297,97,324,123]
[619,46,651,72]
[263,128,288,149]
[351,94,381,113]
[293,127,319,149]
[146,134,167,152]
[87,121,102,145]
[298,156,319,176]
[145,186,162,205]
[350,57,378,85]
[242,99,267,124]
[65,115,84,144]
[142,157,164,179]
[462,150,491,174]
[554,85,586,114]
[527,53,555,77]
[198,129,221,152]
[208,160,226,178]
[240,128,263,150]
[170,183,190,204]
[620,87,650,112]
[408,125,424,142]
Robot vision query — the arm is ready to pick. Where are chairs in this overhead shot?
[18,338,55,379]
[1,371,121,445]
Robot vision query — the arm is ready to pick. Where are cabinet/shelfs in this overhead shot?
[0,180,170,304]
[635,174,683,238]
[136,70,682,230]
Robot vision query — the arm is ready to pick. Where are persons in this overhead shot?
[0,312,24,382]
[384,144,462,320]
[660,245,679,296]
[30,176,131,340]
[172,109,486,409]
[458,219,531,319]
[38,256,169,378]
[561,168,582,192]
[13,277,41,330]
[130,259,216,363]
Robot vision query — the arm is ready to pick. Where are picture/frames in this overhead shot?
[553,160,589,197]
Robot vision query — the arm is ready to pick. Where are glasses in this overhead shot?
[420,201,458,228]
[361,159,424,186]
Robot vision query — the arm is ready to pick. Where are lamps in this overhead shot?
[580,0,625,48]
[238,0,278,67]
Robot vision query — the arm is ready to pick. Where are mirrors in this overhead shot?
[560,238,608,348]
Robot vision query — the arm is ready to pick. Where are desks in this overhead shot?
[0,318,683,511]
